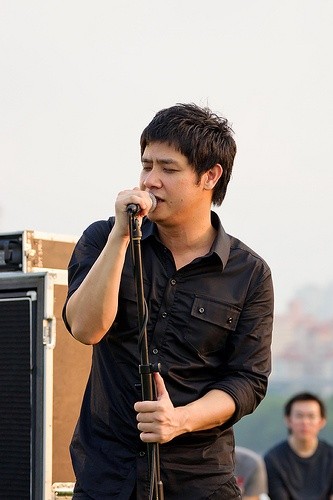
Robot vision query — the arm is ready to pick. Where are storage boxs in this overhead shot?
[0,228,83,277]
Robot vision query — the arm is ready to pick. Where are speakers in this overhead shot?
[0,272,93,500]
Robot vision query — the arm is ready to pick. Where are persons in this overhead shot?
[61,100,274,500]
[232,446,269,500]
[264,393,333,500]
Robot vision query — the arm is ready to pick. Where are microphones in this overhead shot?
[127,191,157,213]
